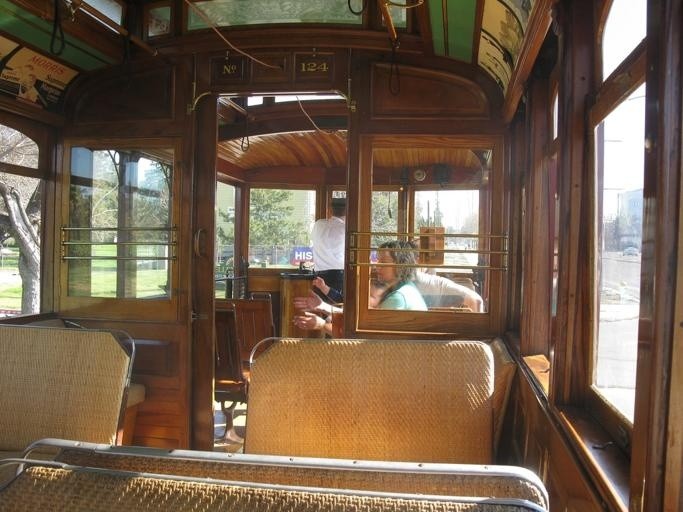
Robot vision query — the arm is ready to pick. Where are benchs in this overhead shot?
[0,437,550,512]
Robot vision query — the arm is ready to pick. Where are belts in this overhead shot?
[316,270,344,275]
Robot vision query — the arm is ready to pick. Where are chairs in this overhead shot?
[234,292,276,363]
[446,277,475,292]
[245,337,496,465]
[484,338,516,457]
[213,301,250,448]
[1,322,137,452]
[429,306,473,313]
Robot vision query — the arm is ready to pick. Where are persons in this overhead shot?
[406,242,483,313]
[312,275,390,309]
[308,198,345,337]
[290,242,428,337]
